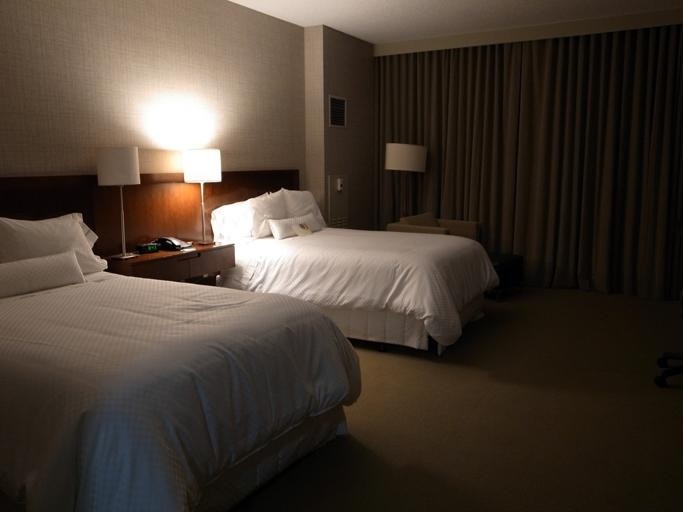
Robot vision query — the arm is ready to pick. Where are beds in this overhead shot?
[0,174,362,512]
[149,169,500,357]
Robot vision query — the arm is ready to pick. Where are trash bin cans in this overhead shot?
[484,252,524,301]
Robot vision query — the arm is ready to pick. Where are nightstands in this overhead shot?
[104,241,235,286]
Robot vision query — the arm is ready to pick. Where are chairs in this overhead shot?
[386,212,481,240]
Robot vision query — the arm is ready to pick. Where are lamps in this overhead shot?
[384,143,428,218]
[97,146,141,261]
[183,149,222,245]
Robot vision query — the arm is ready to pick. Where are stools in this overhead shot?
[653,352,683,388]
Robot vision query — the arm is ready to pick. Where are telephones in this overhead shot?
[152,236,192,249]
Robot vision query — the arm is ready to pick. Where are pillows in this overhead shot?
[0,248,87,297]
[282,187,327,228]
[268,213,320,240]
[0,213,109,275]
[210,192,268,243]
[247,190,285,239]
